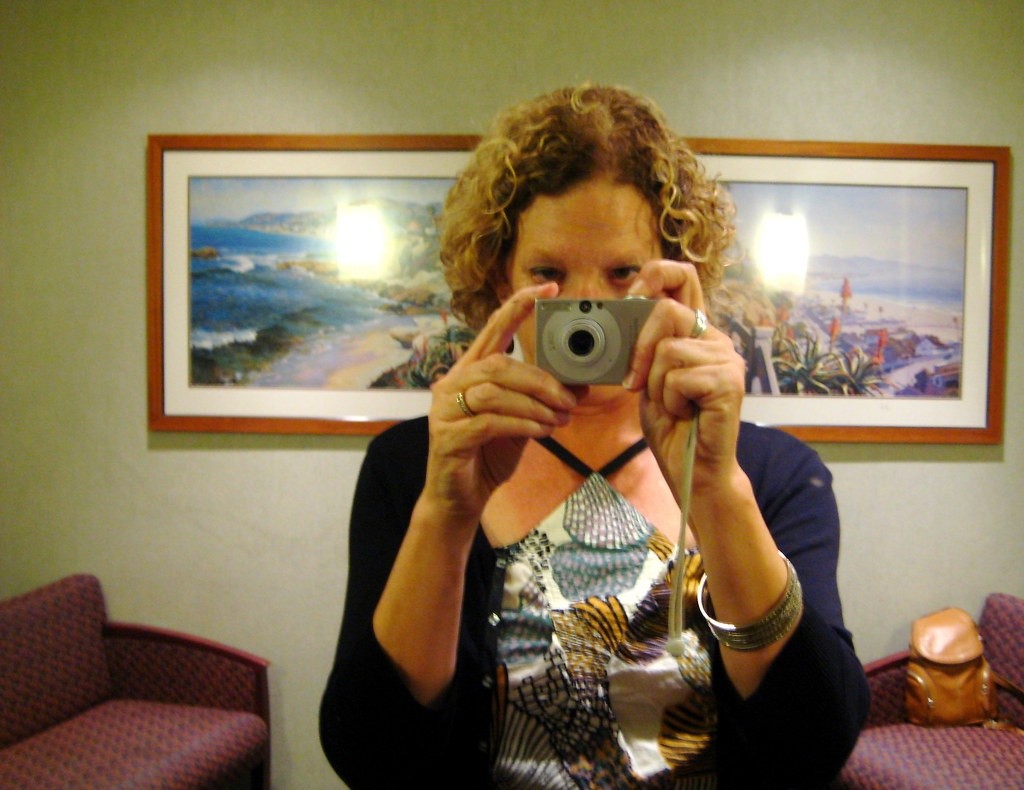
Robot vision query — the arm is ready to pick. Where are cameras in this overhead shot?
[534,297,657,387]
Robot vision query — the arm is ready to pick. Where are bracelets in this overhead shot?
[696,547,803,651]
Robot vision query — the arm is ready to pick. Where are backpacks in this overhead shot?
[904,608,1024,737]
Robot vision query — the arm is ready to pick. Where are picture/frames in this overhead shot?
[145,135,1010,446]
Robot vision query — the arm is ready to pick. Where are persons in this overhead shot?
[319,82,870,790]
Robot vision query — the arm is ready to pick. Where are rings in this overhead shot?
[690,308,707,338]
[457,390,475,417]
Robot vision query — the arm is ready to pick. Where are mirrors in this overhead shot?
[0,0,1024,790]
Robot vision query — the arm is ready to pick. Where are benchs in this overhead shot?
[0,572,277,790]
[843,592,1024,790]
